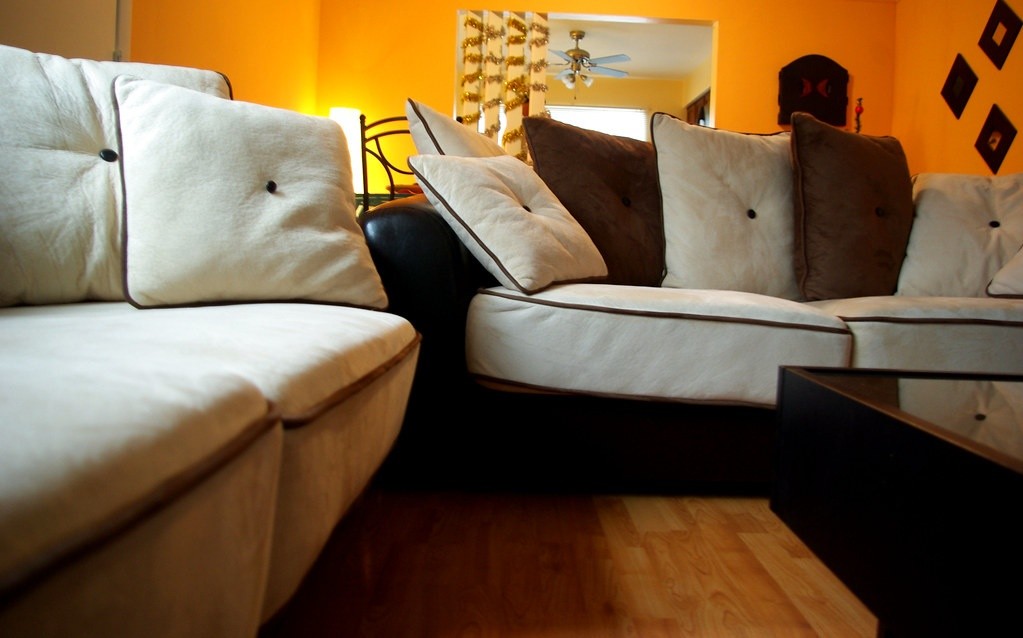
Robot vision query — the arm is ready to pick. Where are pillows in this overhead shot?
[111,73,390,311]
[650,111,800,301]
[522,116,666,288]
[895,172,1023,298]
[0,44,234,307]
[407,155,609,296]
[790,112,915,302]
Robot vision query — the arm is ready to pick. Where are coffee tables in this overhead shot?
[766,365,1023,638]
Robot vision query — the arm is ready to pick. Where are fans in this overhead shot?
[547,30,631,89]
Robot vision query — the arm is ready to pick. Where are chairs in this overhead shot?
[359,114,463,214]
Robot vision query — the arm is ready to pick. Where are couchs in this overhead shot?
[0,300,421,638]
[364,192,1023,496]
